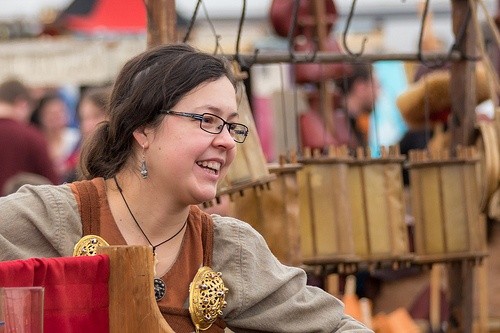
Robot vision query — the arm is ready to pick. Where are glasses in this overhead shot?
[159,109,249,143]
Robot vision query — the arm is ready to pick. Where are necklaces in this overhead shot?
[114,175,188,278]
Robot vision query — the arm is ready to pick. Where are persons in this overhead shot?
[399,57,460,252]
[0,81,112,198]
[329,56,378,158]
[0,44,376,333]
